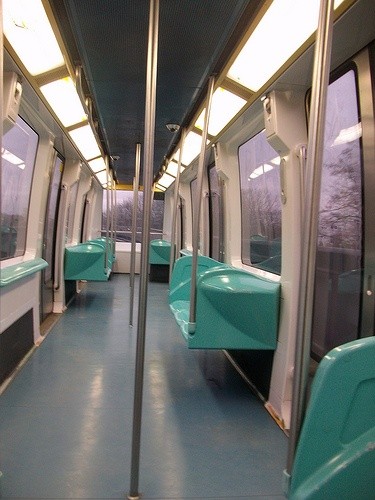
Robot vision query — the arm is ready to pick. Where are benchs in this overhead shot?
[148,239,176,264]
[64,237,116,280]
[168,255,281,351]
